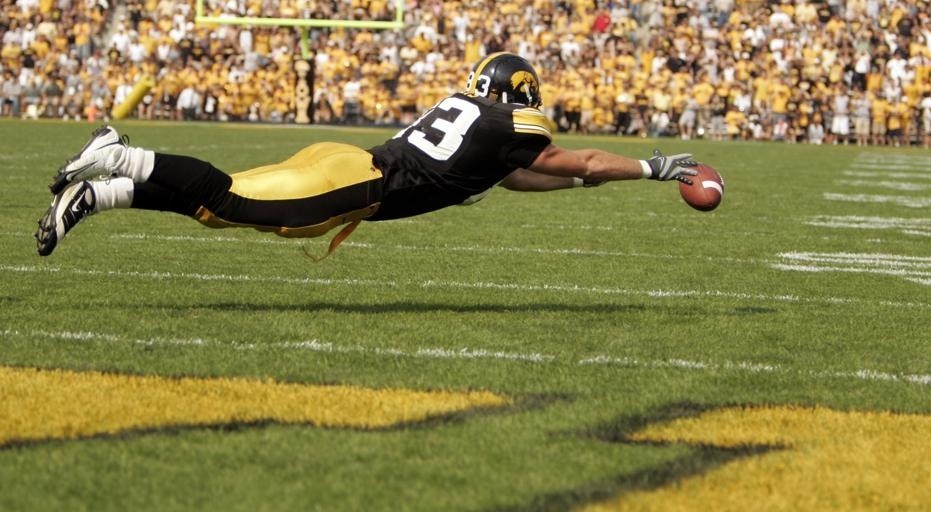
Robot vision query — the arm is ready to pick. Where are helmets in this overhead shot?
[465,51,542,108]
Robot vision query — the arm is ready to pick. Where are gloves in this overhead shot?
[638,148,705,186]
[572,175,608,188]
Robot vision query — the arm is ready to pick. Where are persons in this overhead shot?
[0,1,931,148]
[35,48,703,254]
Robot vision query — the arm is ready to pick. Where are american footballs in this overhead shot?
[679,161,724,209]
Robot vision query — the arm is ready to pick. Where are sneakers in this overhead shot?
[49,124,125,194]
[35,177,99,256]
